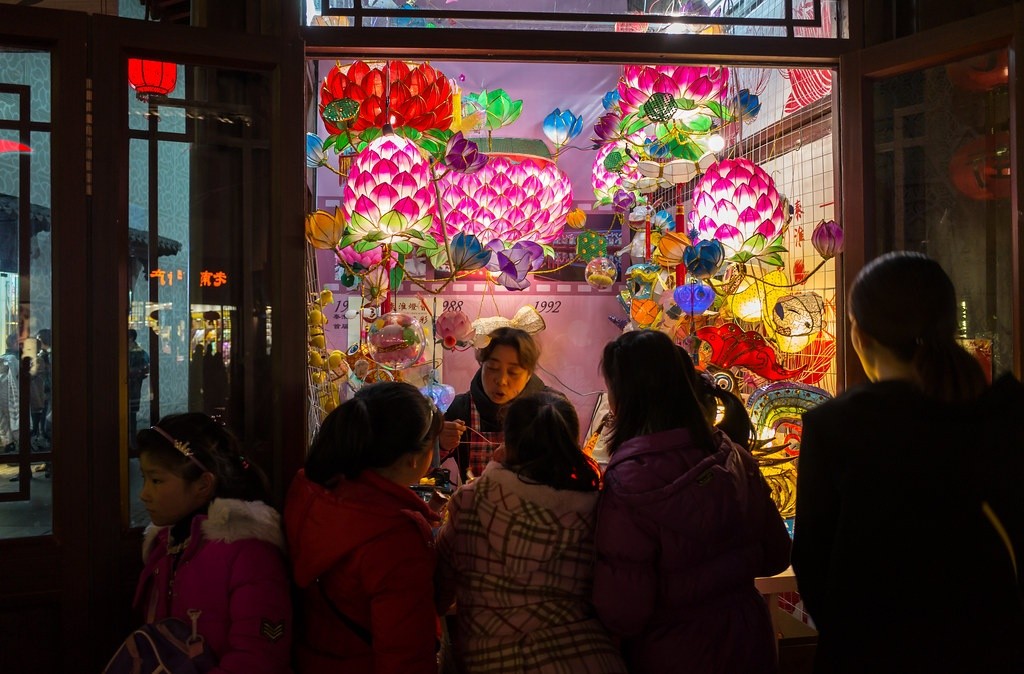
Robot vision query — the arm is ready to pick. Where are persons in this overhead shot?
[128,328,150,458]
[431,327,547,483]
[30,329,52,437]
[130,412,294,674]
[435,388,627,674]
[3,333,19,360]
[792,250,1024,674]
[282,381,444,674]
[591,329,793,674]
[192,344,228,407]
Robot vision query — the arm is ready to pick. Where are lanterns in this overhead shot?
[943,46,1009,95]
[128,58,177,102]
[949,130,1012,201]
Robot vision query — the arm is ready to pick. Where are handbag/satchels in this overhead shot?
[103,617,215,674]
